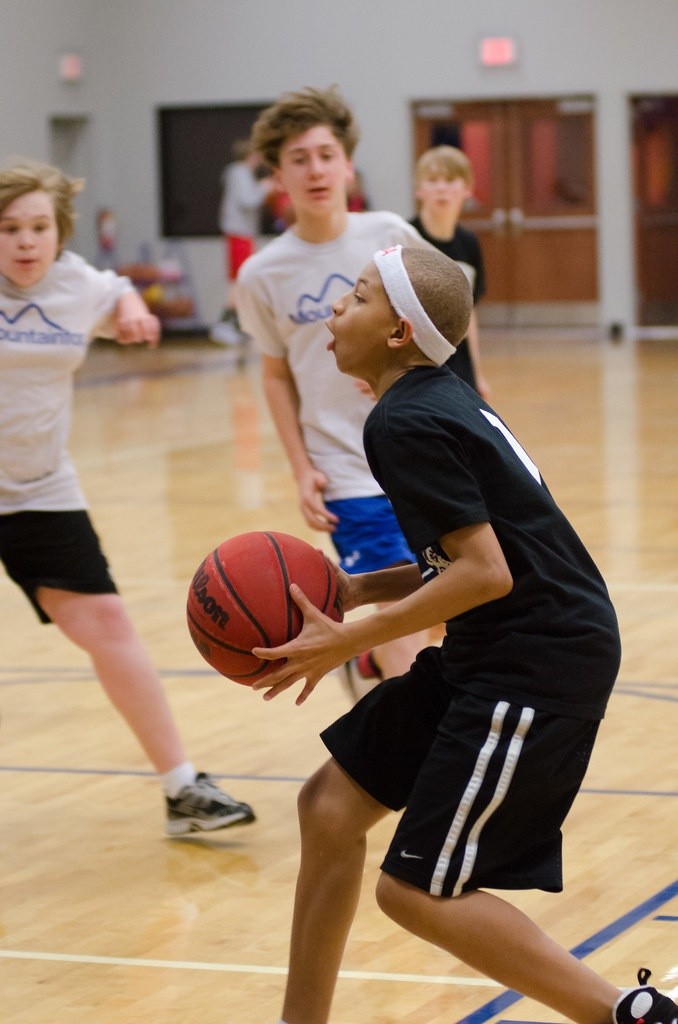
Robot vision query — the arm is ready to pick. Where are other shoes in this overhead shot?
[211,322,247,344]
[612,967,678,1024]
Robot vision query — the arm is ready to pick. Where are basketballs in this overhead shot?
[171,294,196,323]
[267,189,292,217]
[156,257,185,284]
[187,531,343,687]
[126,261,156,285]
[142,286,169,315]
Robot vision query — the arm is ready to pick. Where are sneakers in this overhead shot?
[163,771,256,836]
[338,651,385,703]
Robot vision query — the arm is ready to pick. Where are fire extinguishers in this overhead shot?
[97,207,114,254]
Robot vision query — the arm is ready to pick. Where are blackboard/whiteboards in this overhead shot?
[154,99,288,237]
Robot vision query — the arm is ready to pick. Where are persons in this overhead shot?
[402,145,490,397]
[251,245,678,1023]
[209,133,370,347]
[0,157,257,833]
[232,89,450,702]
